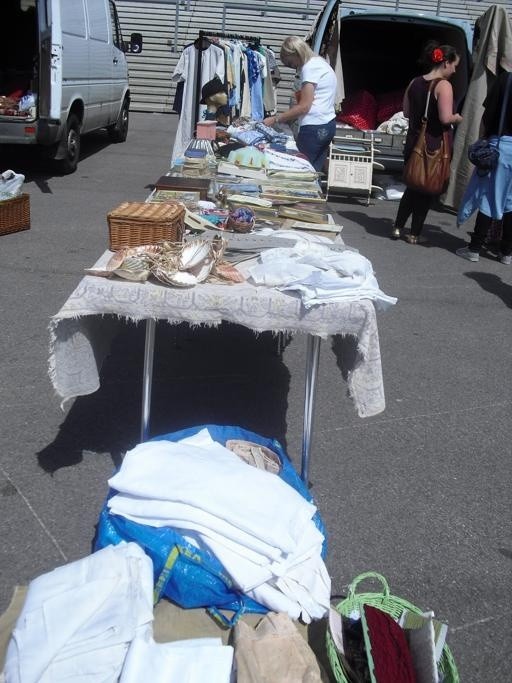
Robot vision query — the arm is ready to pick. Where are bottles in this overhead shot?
[198,78,228,104]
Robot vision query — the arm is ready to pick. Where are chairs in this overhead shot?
[390,225,428,245]
[456,245,481,264]
[500,253,511,264]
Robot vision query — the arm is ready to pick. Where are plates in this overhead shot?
[320,135,385,206]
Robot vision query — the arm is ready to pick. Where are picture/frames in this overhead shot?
[83,135,375,492]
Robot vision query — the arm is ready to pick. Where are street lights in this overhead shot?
[93,420,327,624]
[467,137,501,169]
[401,137,450,194]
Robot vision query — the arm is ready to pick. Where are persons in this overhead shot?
[456,70,512,264]
[261,36,339,172]
[200,78,227,120]
[391,45,463,243]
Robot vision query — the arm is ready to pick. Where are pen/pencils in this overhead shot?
[1,1,143,166]
[288,0,473,193]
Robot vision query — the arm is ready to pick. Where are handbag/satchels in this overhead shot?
[275,114,281,123]
